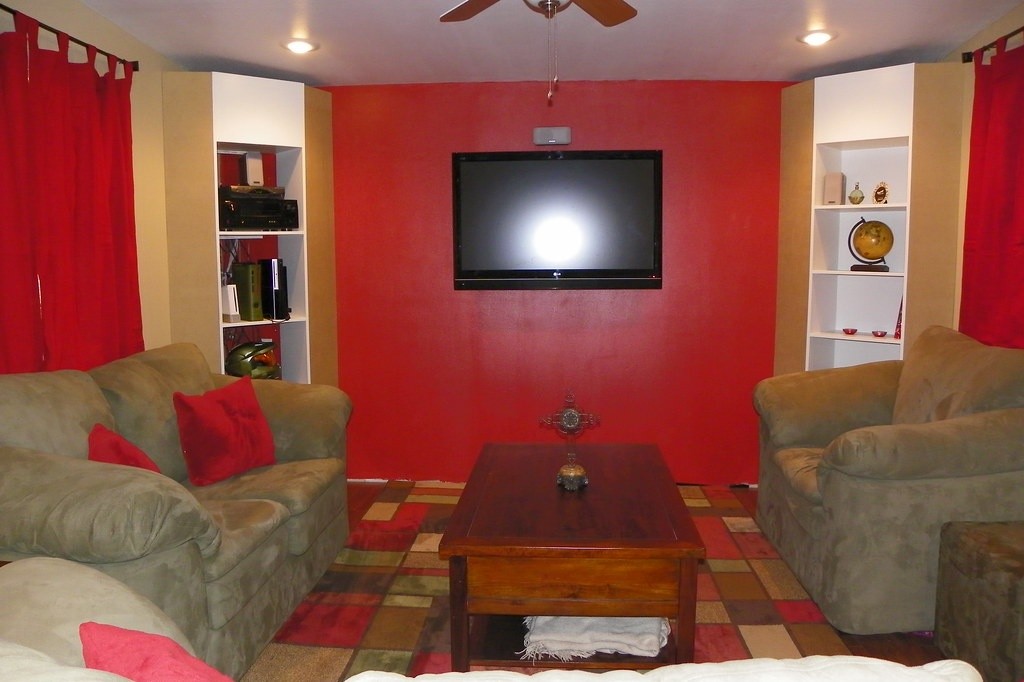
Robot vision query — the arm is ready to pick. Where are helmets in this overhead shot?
[225,342,279,379]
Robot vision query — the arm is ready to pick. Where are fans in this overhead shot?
[440,0,637,26]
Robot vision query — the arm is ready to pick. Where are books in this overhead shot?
[222,284,241,323]
[258,259,288,320]
[231,263,264,322]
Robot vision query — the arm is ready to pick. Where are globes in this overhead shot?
[847,214,895,272]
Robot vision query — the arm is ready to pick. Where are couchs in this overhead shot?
[0,556,985,682]
[753,326,1024,635]
[0,342,353,682]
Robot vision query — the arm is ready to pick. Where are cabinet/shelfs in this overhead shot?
[211,72,311,385]
[804,62,916,372]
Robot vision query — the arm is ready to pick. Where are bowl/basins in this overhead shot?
[872,331,887,337]
[843,328,857,335]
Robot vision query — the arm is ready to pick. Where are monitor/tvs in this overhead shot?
[453,151,663,290]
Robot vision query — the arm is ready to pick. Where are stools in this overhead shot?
[933,520,1024,682]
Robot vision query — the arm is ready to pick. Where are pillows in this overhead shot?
[79,621,234,682]
[173,375,276,486]
[88,423,160,474]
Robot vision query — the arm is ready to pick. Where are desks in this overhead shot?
[438,443,705,673]
